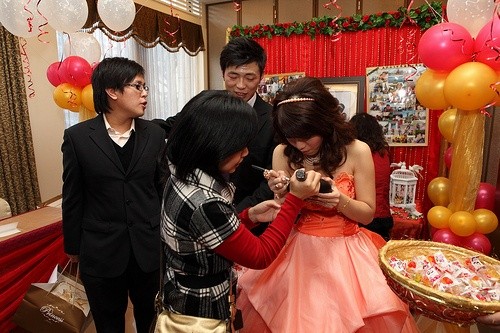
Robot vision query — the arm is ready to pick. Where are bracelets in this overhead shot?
[337,198,351,214]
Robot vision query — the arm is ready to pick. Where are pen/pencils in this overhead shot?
[252,164,291,182]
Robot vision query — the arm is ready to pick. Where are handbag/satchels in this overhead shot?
[12,257,90,333]
[229,305,243,331]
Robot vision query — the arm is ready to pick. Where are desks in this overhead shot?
[0,206,71,293]
[388,200,424,241]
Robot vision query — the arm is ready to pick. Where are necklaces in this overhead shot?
[303,152,321,165]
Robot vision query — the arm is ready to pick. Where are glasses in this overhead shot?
[122,83,149,92]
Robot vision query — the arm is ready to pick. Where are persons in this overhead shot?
[233,75,416,333]
[220,36,281,212]
[344,113,396,242]
[151,90,323,333]
[61,58,174,333]
[477,310,500,331]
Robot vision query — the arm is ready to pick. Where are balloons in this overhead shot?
[0,0,139,112]
[413,15,500,254]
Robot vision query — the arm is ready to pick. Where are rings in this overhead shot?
[263,169,271,180]
[275,184,279,189]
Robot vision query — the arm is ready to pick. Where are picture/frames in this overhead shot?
[364,64,429,146]
[255,72,306,107]
[320,76,365,122]
